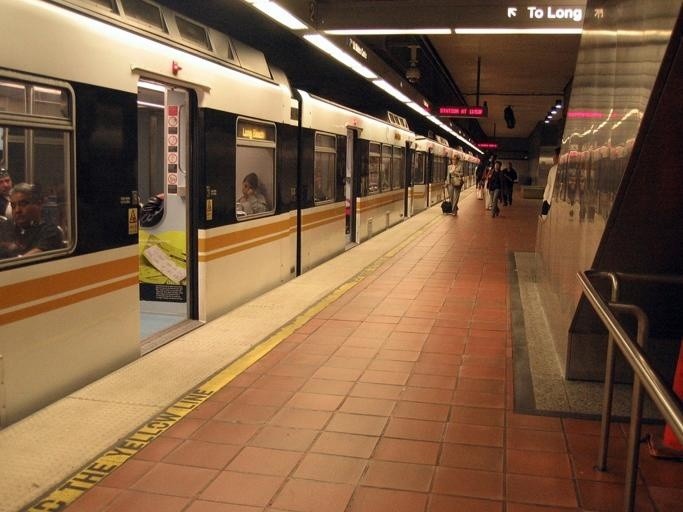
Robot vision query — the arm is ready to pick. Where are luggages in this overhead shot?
[441,186,452,213]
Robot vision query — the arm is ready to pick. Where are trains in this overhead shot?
[552,138,636,221]
[0,1,487,429]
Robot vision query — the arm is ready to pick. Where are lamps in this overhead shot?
[544,99,562,124]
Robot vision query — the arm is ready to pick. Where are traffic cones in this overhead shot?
[645,341,682,461]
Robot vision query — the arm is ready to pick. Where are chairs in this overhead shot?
[0,212,65,253]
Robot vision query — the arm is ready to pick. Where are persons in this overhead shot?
[540,145,561,223]
[236,173,268,216]
[0,181,65,259]
[0,167,14,221]
[442,154,464,216]
[475,155,517,218]
[140,191,166,228]
